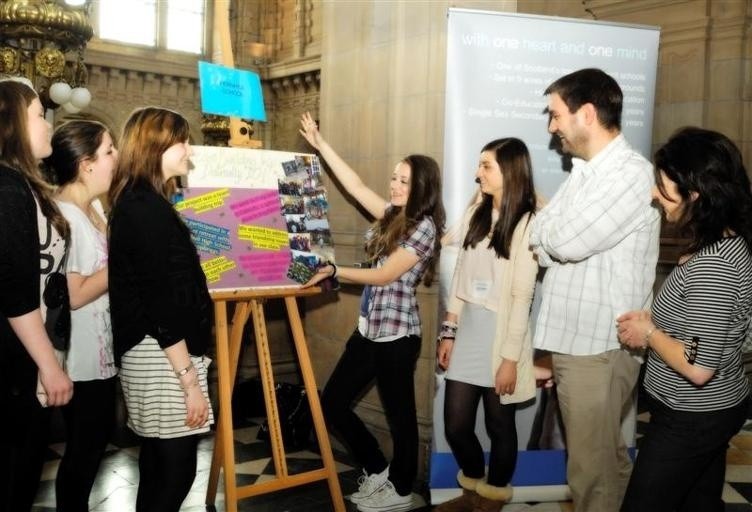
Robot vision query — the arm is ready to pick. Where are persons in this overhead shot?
[105,109,218,510]
[0,79,73,510]
[435,138,542,512]
[296,111,448,512]
[526,68,665,512]
[612,123,752,512]
[36,121,119,512]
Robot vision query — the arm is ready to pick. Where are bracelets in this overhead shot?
[327,264,339,279]
[437,319,457,340]
[642,325,657,350]
[172,363,195,377]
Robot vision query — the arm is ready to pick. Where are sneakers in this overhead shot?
[435,469,514,512]
[352,464,413,512]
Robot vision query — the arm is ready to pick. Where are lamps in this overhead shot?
[48,71,91,114]
